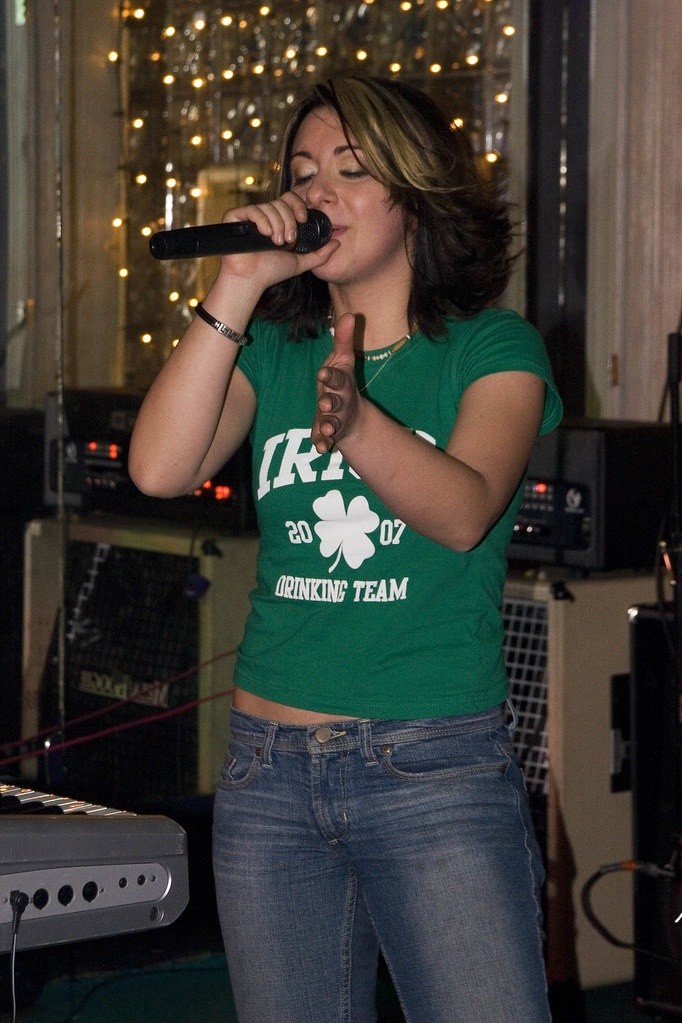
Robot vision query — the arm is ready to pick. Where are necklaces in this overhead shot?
[327,300,413,389]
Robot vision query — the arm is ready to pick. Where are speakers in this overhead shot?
[17,513,262,798]
[496,570,662,990]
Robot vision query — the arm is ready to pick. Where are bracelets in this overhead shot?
[193,300,248,346]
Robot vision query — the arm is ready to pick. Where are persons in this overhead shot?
[130,70,556,1023]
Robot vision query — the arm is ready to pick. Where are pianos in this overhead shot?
[0,782,194,956]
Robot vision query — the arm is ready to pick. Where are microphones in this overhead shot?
[148,209,333,260]
